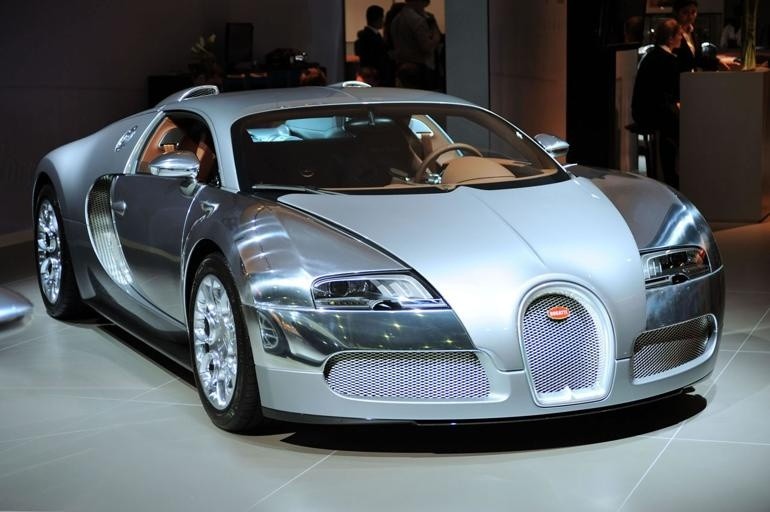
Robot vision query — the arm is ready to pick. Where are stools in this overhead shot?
[625,123,661,177]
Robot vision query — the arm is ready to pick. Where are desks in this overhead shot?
[147,63,326,108]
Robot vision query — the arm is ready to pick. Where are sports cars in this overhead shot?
[33,78,728,431]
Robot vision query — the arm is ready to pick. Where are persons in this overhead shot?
[383,2,409,48]
[353,3,398,88]
[718,14,739,49]
[389,0,444,92]
[670,0,742,72]
[735,25,742,51]
[630,17,718,191]
